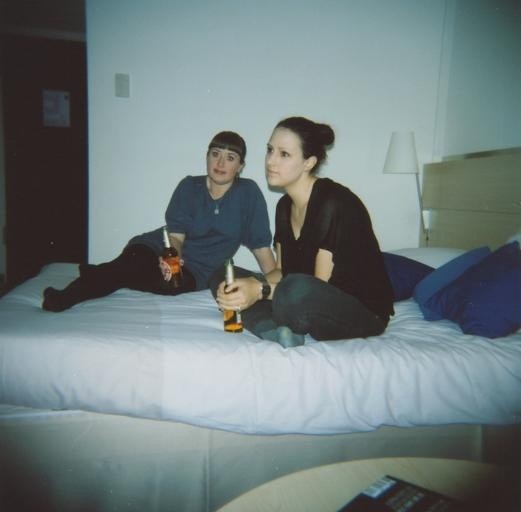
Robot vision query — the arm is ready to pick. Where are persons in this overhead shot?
[210,117,394,347]
[41,131,277,312]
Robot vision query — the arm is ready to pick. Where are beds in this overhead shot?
[0,149,520,512]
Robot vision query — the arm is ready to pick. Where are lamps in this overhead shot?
[381,128,432,243]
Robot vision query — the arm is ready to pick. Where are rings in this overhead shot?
[236,306,239,311]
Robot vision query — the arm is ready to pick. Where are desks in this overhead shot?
[216,457,521,512]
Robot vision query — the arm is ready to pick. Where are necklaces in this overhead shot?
[208,178,230,216]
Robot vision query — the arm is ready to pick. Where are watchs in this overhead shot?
[260,279,270,301]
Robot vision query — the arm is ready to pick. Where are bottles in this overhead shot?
[161,227,185,290]
[224,257,243,333]
